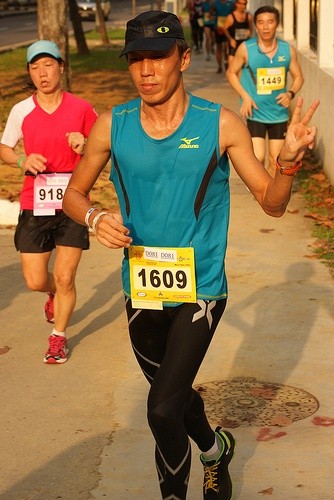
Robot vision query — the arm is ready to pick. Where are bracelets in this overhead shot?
[17,156,25,168]
[274,155,303,176]
[85,208,107,233]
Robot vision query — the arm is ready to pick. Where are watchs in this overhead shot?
[288,91,295,99]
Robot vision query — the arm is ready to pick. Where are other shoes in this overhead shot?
[194,45,228,74]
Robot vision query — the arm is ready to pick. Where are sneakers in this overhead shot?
[199,426,236,500]
[43,333,70,364]
[42,291,55,324]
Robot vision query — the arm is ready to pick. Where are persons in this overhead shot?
[187,0,253,74]
[0,40,100,365]
[226,7,304,178]
[61,10,320,500]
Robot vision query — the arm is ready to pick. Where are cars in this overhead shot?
[75,0,112,22]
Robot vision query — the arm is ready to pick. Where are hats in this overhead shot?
[122,8,186,59]
[27,40,62,63]
